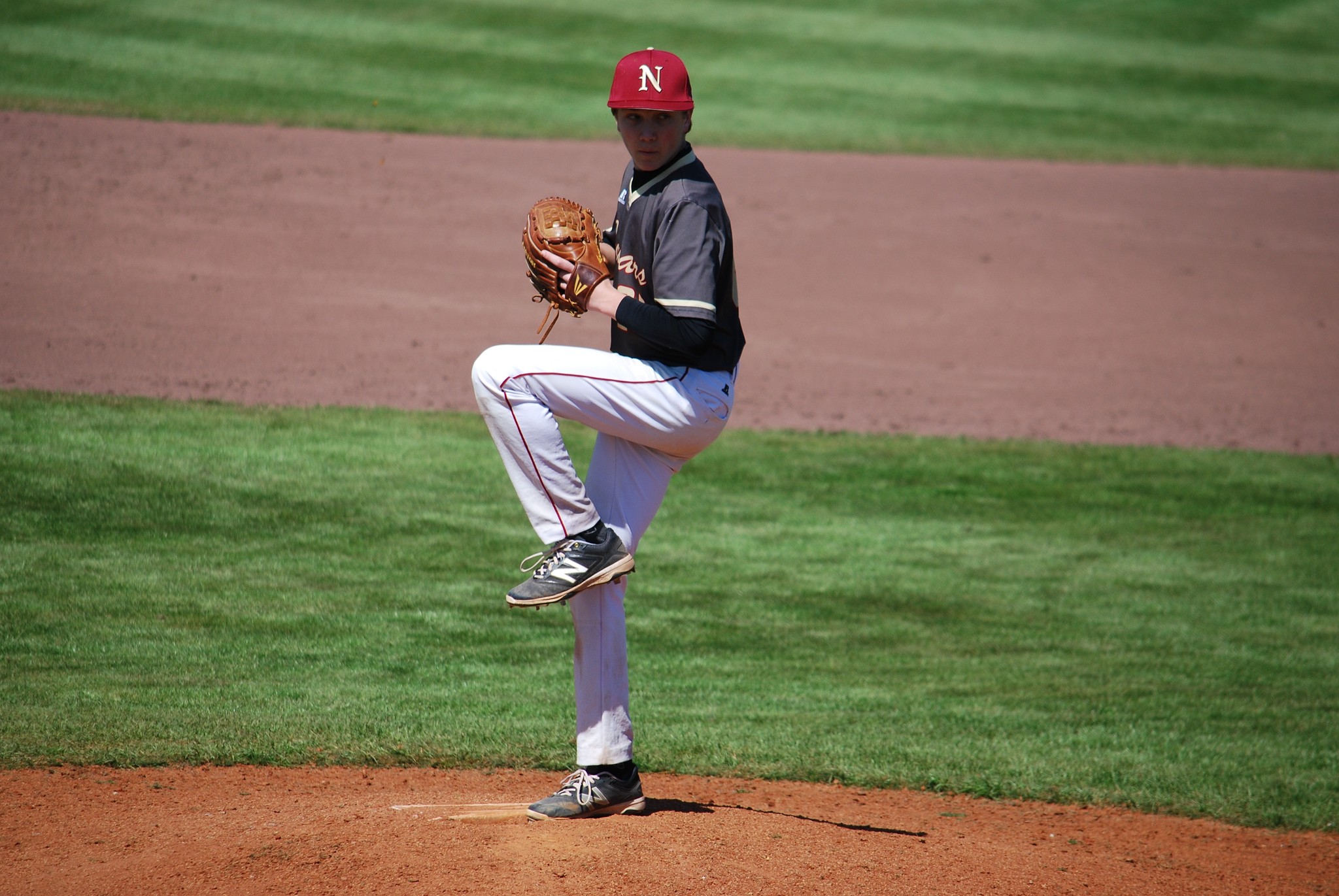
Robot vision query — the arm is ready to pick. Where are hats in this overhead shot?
[608,48,694,111]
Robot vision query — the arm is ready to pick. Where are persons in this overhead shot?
[469,49,746,823]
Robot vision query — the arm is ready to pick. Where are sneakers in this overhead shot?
[526,764,648,821]
[512,527,634,611]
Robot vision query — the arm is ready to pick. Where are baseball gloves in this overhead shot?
[521,197,611,317]
